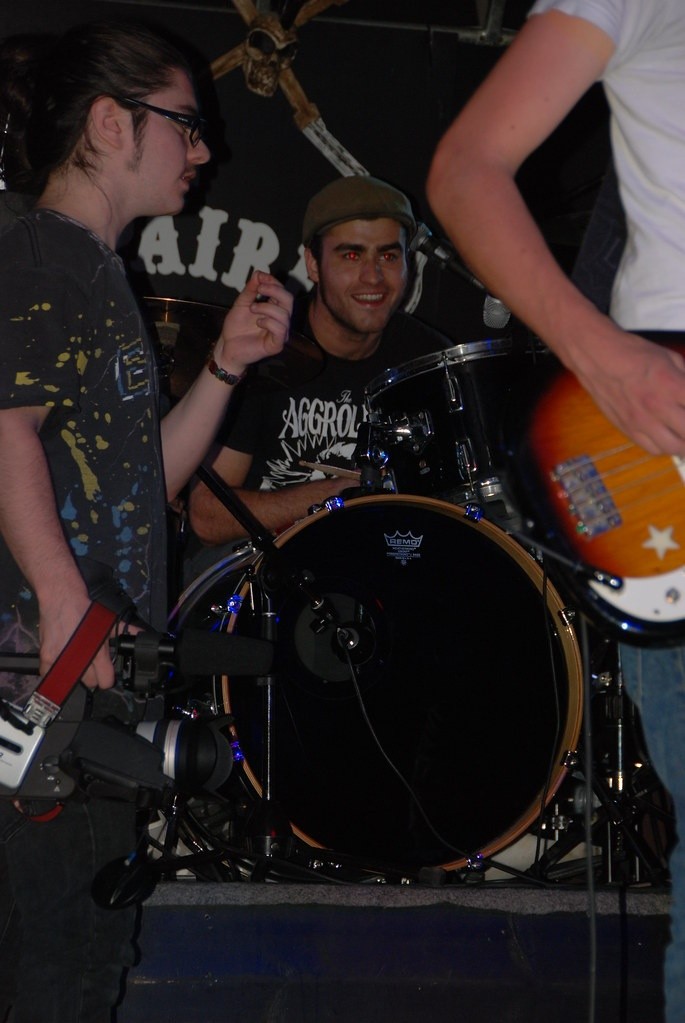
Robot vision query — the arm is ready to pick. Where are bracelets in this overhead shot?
[207,354,244,387]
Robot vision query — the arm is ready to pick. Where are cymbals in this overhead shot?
[137,295,327,397]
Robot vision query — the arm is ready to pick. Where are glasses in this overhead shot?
[87,90,206,148]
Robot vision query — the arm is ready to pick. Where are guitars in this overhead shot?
[502,328,685,648]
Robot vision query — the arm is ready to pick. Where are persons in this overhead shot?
[0,37,290,1022]
[427,0,685,1022]
[193,180,601,882]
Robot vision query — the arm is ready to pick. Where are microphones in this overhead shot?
[309,595,371,660]
[117,628,271,677]
[415,221,512,329]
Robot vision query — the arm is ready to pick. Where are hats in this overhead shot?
[301,175,418,249]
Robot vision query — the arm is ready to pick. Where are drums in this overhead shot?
[361,332,561,512]
[166,486,585,878]
